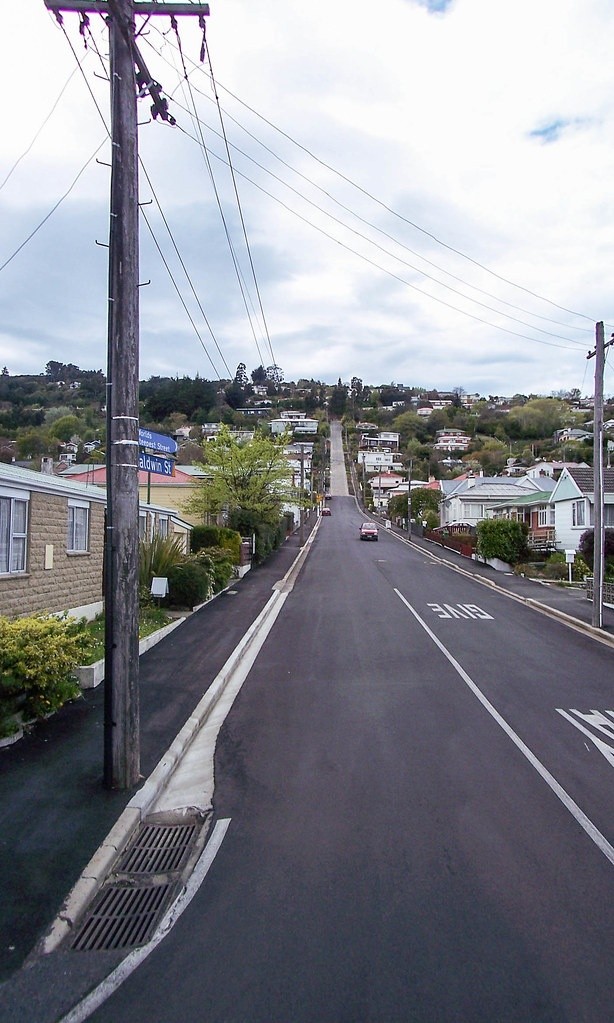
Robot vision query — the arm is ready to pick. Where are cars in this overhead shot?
[321,508,332,516]
[359,521,380,541]
[325,494,332,500]
